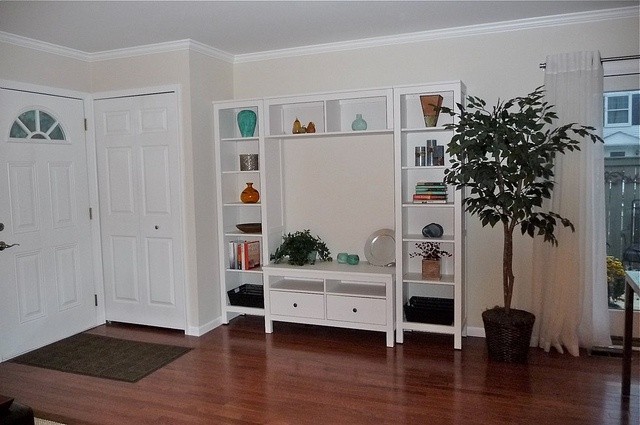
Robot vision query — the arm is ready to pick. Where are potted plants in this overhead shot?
[269,226,332,265]
[429,83,604,365]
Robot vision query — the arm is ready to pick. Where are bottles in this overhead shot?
[414,146,425,166]
[351,113,367,131]
[427,139,437,166]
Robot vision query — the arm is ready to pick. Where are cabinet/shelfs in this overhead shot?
[211,96,274,334]
[394,80,467,351]
[262,86,396,348]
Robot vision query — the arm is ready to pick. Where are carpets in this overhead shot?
[4,333,193,383]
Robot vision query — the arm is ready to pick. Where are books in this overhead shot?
[413,182,448,203]
[229,240,260,270]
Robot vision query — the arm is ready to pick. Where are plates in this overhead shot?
[364,229,395,266]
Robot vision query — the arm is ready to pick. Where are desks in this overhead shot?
[621,270,640,397]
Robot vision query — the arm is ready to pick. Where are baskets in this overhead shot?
[403,295,454,325]
[227,283,264,308]
